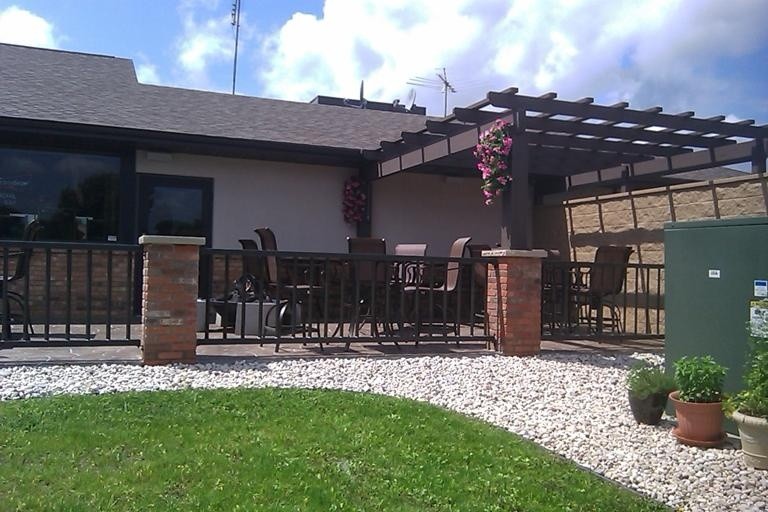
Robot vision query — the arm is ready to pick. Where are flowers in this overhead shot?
[338,174,370,228]
[461,117,512,205]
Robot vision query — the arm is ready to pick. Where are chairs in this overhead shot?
[237,226,492,341]
[543,245,635,336]
[1,216,51,342]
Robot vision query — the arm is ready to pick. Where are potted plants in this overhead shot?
[719,295,767,471]
[665,352,730,449]
[621,352,677,425]
